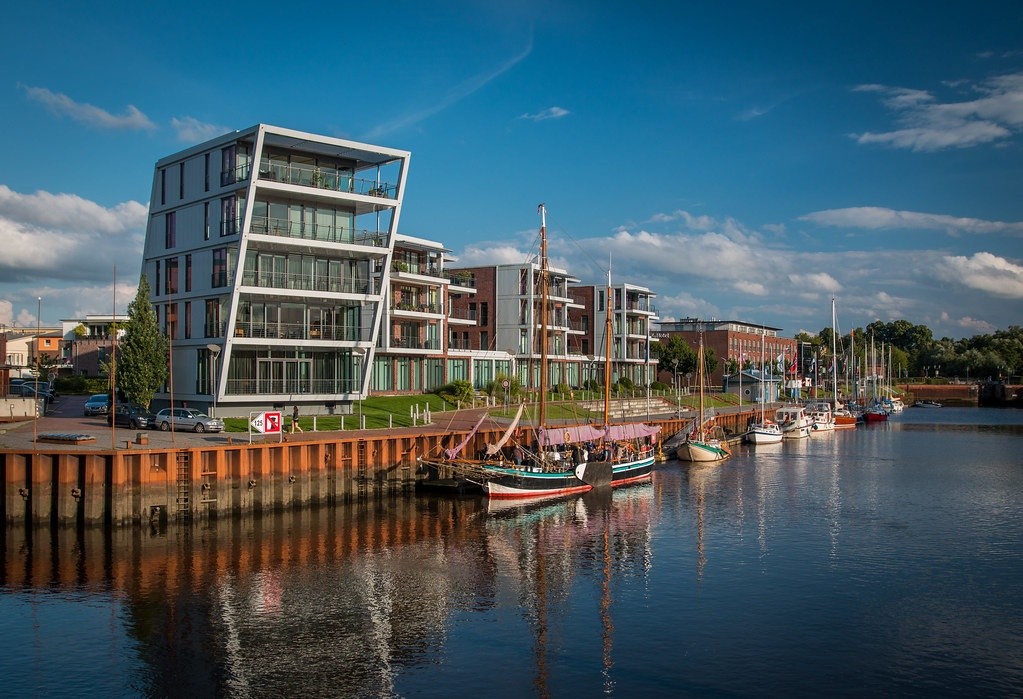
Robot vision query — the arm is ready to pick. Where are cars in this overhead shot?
[10,379,56,403]
[109,402,157,429]
[84,394,109,416]
[155,407,225,433]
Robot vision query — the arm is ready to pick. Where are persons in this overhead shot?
[290,406,303,434]
[478,441,495,460]
[511,440,646,466]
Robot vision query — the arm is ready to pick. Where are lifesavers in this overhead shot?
[564,432,572,443]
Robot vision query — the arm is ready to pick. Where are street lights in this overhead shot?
[720,357,731,393]
[207,344,221,418]
[587,355,595,402]
[352,347,366,428]
[672,359,679,392]
[506,349,515,379]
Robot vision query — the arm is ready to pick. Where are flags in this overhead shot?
[790,352,797,373]
[809,354,815,372]
[644,333,648,365]
[828,358,833,372]
[777,353,783,372]
[841,357,847,373]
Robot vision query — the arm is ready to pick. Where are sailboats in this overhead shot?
[806,352,835,431]
[674,325,732,462]
[830,298,905,426]
[417,203,661,498]
[745,330,784,444]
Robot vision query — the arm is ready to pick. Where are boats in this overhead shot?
[775,405,815,439]
[978,376,1009,408]
[916,399,942,408]
